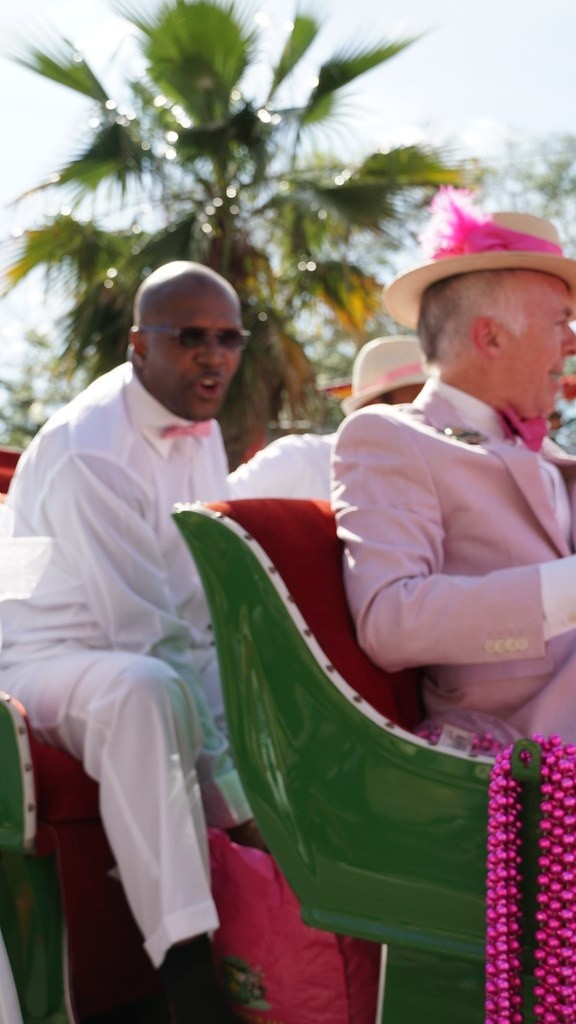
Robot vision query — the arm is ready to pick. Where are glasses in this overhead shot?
[143,328,251,351]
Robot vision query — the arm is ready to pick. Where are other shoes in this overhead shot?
[160,942,239,1024]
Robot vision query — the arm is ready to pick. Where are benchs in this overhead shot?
[0,448,543,1024]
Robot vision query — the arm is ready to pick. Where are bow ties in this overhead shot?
[495,405,546,452]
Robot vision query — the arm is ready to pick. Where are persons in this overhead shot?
[332,190,576,747]
[228,333,430,503]
[0,261,265,1024]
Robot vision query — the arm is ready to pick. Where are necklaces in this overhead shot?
[430,725,576,1024]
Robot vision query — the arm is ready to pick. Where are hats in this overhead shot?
[383,185,576,336]
[340,333,427,417]
